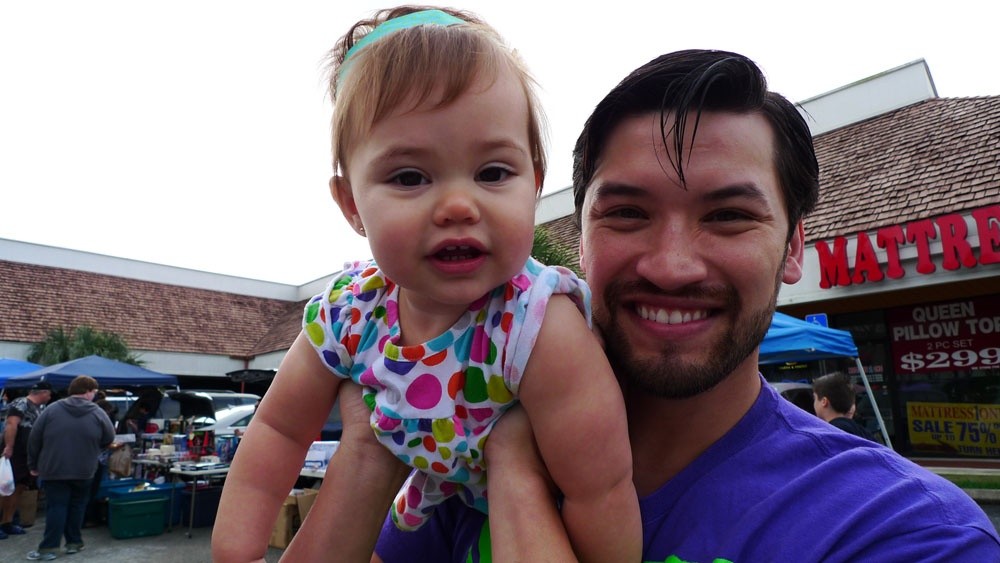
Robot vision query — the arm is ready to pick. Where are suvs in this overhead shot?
[101,388,260,456]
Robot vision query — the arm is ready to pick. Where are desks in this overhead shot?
[132,458,167,478]
[167,463,229,539]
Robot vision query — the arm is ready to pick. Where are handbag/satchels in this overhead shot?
[0,457,15,496]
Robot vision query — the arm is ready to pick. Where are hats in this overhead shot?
[31,382,57,392]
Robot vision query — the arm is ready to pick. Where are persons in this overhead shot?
[0,381,52,539]
[812,372,879,442]
[211,5,642,563]
[27,376,115,561]
[276,50,1000,563]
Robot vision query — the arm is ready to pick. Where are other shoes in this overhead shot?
[0,529,7,539]
[66,542,85,553]
[26,550,56,560]
[4,525,26,535]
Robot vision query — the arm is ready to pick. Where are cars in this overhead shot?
[859,385,946,442]
[767,382,817,417]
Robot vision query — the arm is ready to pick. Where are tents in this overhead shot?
[0,358,45,388]
[758,312,893,449]
[4,354,180,392]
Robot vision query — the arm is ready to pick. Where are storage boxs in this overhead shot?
[96,477,221,540]
[268,489,319,549]
